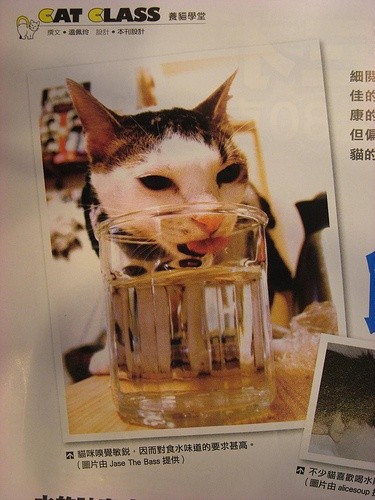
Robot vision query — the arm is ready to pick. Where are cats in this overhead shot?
[65,67,292,376]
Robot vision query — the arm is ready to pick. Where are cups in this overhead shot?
[93,202,275,429]
